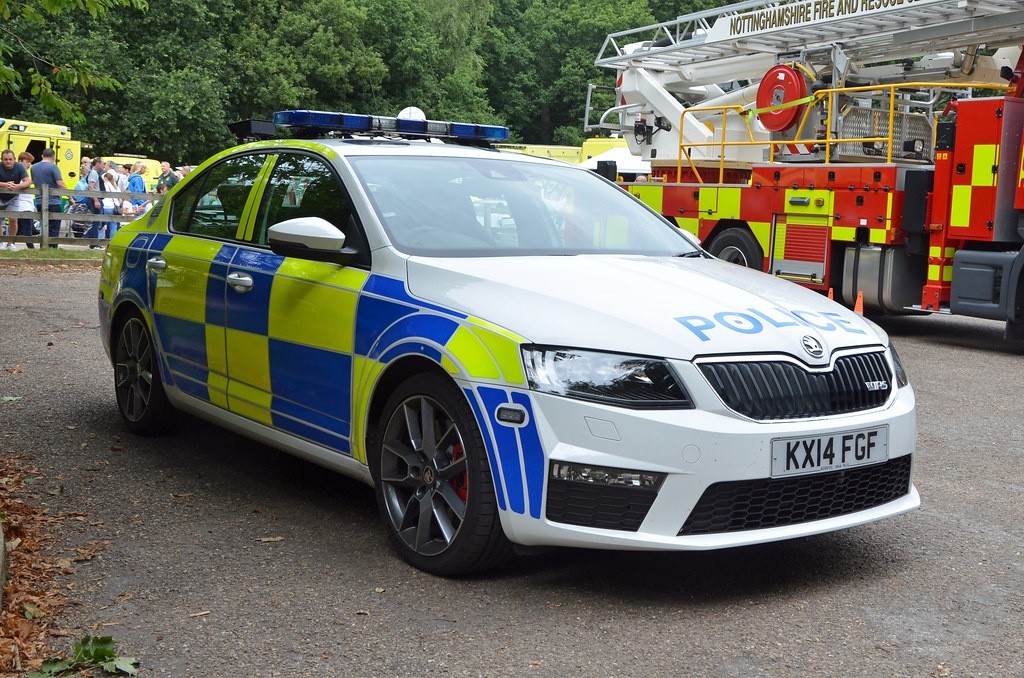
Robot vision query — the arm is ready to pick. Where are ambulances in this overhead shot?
[98,110,921,579]
[79,153,163,193]
[0,118,81,225]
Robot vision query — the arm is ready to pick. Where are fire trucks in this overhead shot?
[577,0,1024,323]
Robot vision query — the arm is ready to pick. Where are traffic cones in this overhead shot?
[827,287,833,300]
[853,290,863,316]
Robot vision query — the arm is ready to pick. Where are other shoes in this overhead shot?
[90,245,105,251]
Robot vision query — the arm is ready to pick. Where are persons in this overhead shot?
[0,148,193,251]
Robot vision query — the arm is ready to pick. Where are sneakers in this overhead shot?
[0,243,18,251]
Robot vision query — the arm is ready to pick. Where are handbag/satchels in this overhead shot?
[73,170,99,202]
[114,205,123,215]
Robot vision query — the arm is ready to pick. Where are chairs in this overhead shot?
[409,180,497,246]
[217,183,273,238]
[301,176,353,262]
